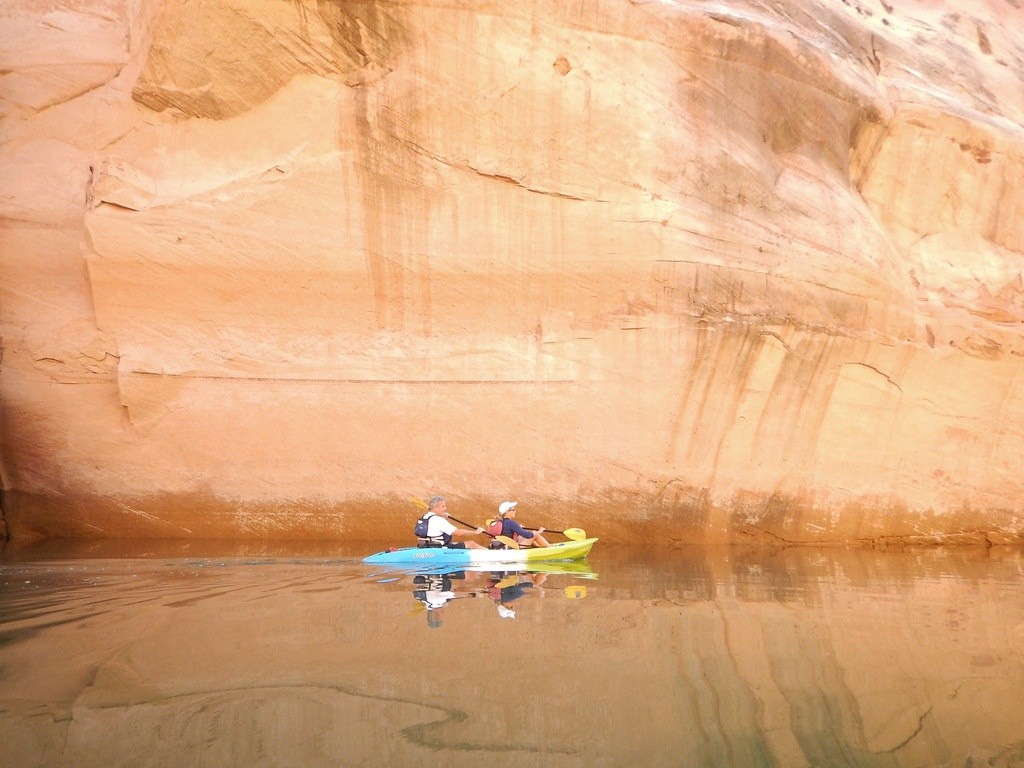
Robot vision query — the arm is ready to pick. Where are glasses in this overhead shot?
[509,508,516,511]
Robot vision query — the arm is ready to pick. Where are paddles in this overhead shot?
[493,575,587,599]
[485,519,586,541]
[410,597,467,616]
[410,497,520,550]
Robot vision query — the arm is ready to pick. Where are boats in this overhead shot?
[361,537,599,564]
[368,557,600,579]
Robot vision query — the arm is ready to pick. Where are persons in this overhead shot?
[414,495,486,549]
[486,571,547,618]
[488,501,551,550]
[411,574,484,628]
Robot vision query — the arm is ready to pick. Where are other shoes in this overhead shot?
[488,541,505,550]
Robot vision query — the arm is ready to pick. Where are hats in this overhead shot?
[497,605,515,619]
[499,502,518,516]
